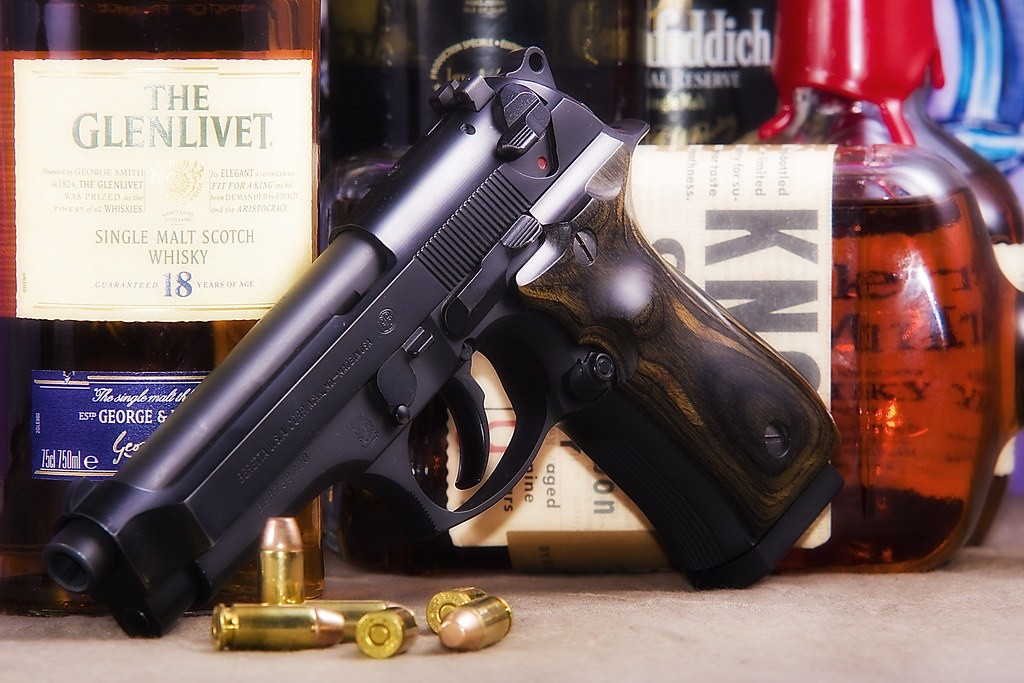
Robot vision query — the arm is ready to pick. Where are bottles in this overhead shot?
[321,1,1023,573]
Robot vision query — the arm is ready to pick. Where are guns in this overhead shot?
[41,42,848,642]
